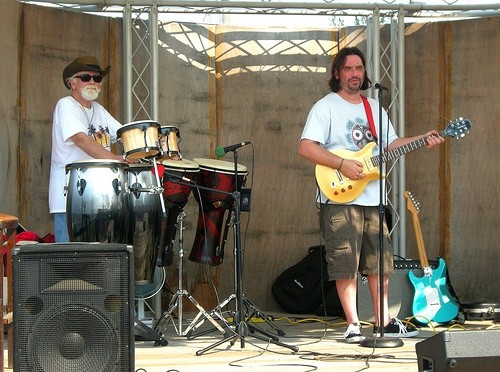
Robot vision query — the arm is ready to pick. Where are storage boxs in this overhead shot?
[464,302,500,319]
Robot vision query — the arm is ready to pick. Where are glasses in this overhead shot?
[73,74,103,83]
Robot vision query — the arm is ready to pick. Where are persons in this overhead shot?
[298,47,444,343]
[49,56,137,316]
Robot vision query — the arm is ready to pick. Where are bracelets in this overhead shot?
[338,159,344,170]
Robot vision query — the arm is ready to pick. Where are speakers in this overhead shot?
[12,244,136,372]
[357,259,438,325]
[415,329,500,372]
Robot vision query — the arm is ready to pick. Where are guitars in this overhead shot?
[315,116,472,203]
[403,191,460,324]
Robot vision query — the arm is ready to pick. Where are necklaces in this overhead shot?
[81,105,94,124]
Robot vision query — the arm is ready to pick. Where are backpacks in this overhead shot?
[272,245,346,320]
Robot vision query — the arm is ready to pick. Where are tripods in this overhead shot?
[154,152,299,355]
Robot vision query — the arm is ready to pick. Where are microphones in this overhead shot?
[215,142,251,157]
[372,81,388,90]
[151,165,195,184]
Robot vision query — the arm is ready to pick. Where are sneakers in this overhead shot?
[373,318,420,337]
[343,322,366,342]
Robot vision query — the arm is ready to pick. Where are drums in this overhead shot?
[188,158,249,266]
[158,126,181,159]
[117,120,162,163]
[128,163,162,286]
[153,158,199,266]
[65,159,135,246]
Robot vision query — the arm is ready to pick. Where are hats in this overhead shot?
[62,56,111,90]
